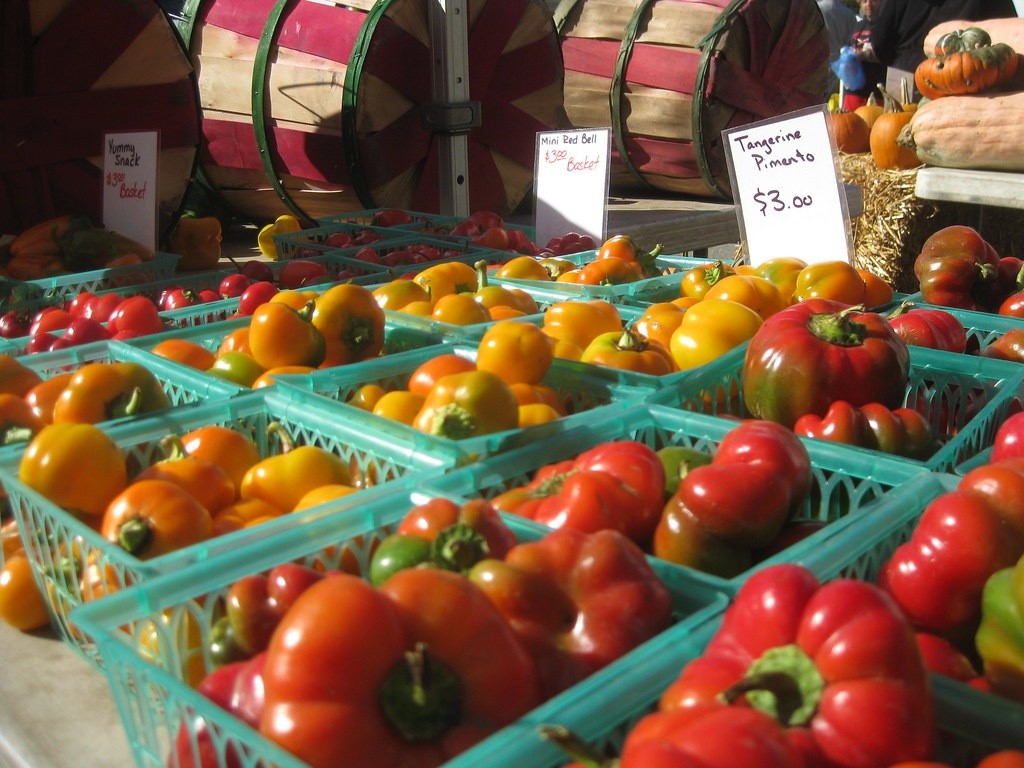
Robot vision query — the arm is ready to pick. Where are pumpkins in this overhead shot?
[826,16,1024,173]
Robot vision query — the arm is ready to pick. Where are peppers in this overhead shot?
[0,209,1024,768]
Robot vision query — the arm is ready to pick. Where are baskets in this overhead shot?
[0,210,1024,768]
